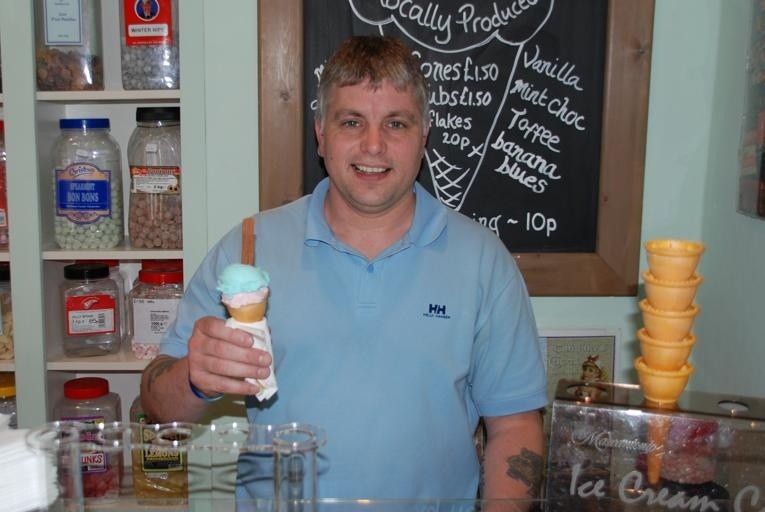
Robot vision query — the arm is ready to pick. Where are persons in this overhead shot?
[136,31,549,511]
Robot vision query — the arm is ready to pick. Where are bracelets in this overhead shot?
[185,368,225,403]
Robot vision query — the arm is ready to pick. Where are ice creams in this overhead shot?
[214,217,270,322]
[349,0,554,211]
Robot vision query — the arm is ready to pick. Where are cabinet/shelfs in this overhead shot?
[0,0,211,472]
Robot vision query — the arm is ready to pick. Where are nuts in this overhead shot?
[122,41,179,90]
[37,48,102,91]
[129,193,182,249]
[51,166,124,249]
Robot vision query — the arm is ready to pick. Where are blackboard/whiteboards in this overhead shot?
[257,0,656,296]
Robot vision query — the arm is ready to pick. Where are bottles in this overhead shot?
[35,0,103,92]
[55,376,124,501]
[117,0,179,91]
[50,116,125,252]
[59,261,125,358]
[125,257,183,361]
[130,388,183,501]
[127,104,183,252]
